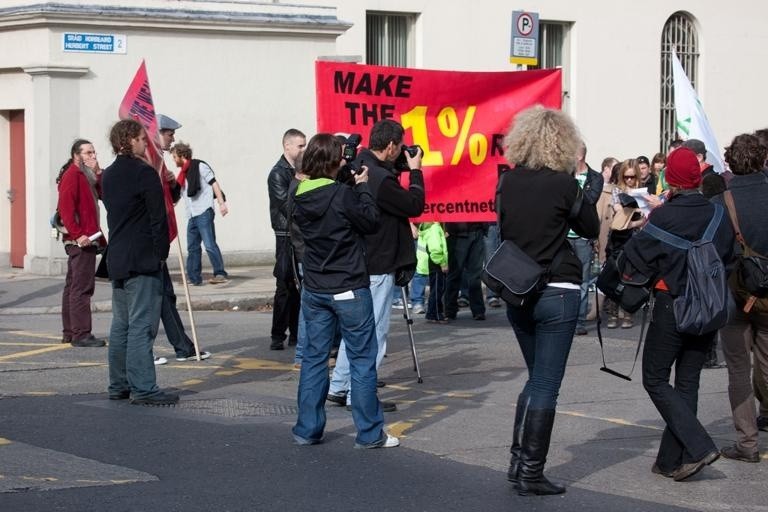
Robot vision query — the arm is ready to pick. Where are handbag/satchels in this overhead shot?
[596,250,654,314]
[728,248,768,317]
[479,240,548,308]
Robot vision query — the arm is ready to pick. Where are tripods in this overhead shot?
[382,282,425,384]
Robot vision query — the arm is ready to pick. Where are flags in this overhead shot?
[312,58,563,222]
[115,60,178,242]
[669,48,728,174]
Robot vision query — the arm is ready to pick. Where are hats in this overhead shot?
[665,147,702,189]
[156,114,182,130]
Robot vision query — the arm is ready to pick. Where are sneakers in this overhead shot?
[391,293,635,335]
[270,334,400,448]
[207,277,228,283]
[757,415,768,431]
[107,351,211,406]
[71,335,106,347]
[721,446,760,462]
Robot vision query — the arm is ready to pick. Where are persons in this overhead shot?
[290,132,405,449]
[622,147,721,482]
[478,103,602,496]
[170,143,230,286]
[266,127,347,369]
[391,220,501,325]
[567,129,768,369]
[709,133,768,464]
[100,118,180,404]
[325,119,425,411]
[57,140,107,347]
[153,111,212,365]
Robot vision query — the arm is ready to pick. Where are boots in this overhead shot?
[507,392,566,496]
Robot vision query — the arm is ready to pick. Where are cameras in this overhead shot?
[339,133,364,184]
[399,142,420,164]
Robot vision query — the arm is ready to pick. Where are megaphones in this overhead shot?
[49,209,79,235]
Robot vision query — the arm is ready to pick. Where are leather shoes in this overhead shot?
[673,448,721,481]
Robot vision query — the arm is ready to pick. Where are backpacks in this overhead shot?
[641,201,736,337]
